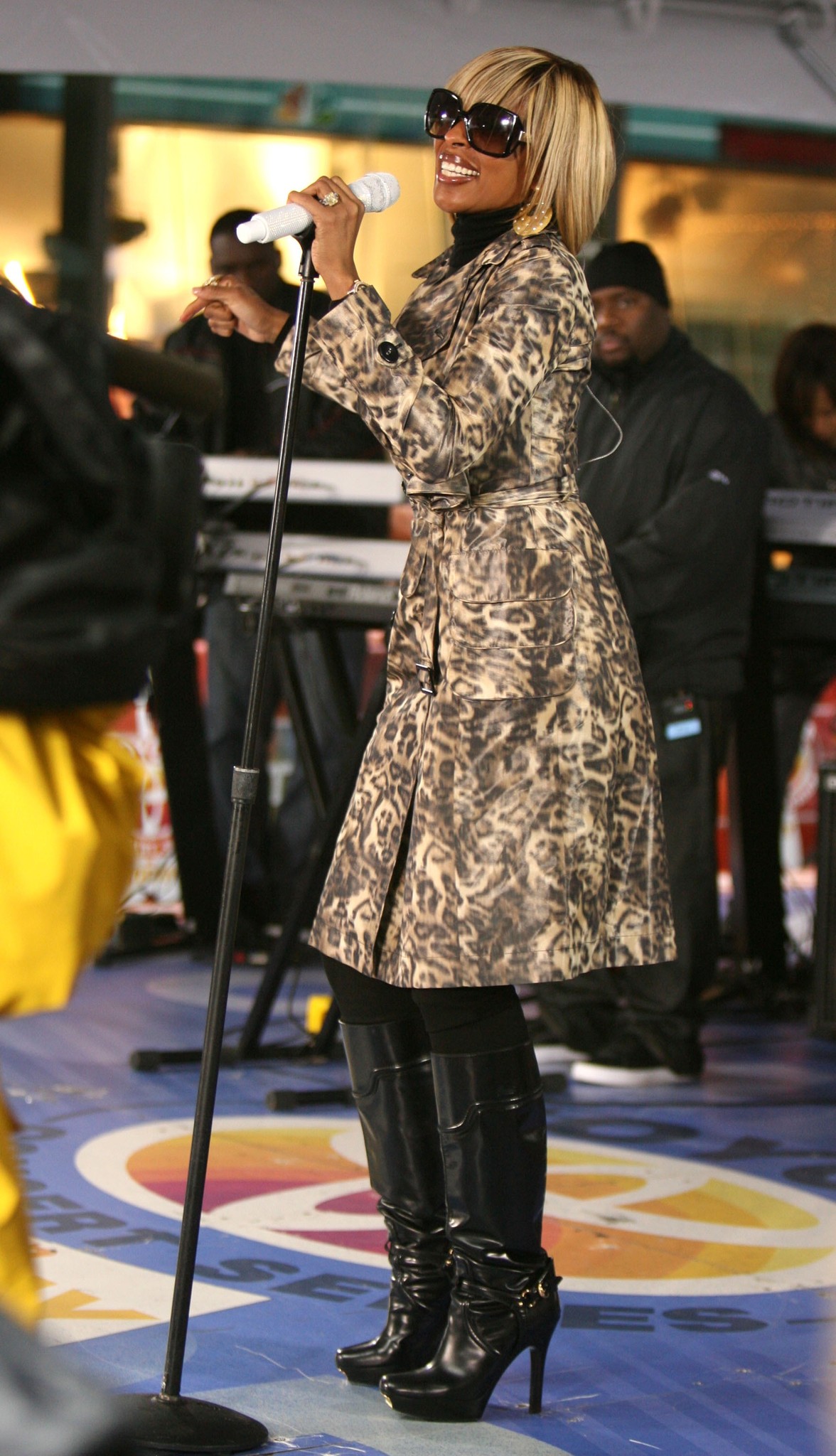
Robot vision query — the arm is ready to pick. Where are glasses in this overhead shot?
[425,88,532,157]
[214,255,266,273]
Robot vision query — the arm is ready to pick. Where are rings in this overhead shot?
[319,192,339,207]
[201,274,223,288]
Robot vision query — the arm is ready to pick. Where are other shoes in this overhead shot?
[246,923,282,965]
[570,1051,698,1088]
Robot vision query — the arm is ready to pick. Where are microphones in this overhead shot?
[237,172,402,244]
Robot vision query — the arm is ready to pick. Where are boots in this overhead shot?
[336,1019,451,1384]
[380,1040,560,1422]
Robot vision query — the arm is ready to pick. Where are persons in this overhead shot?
[0,209,836,1456]
[180,47,679,1421]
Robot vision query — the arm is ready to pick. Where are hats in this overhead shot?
[584,242,669,308]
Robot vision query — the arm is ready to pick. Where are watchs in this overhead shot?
[329,279,369,310]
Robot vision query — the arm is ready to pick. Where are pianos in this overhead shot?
[126,456,573,1115]
